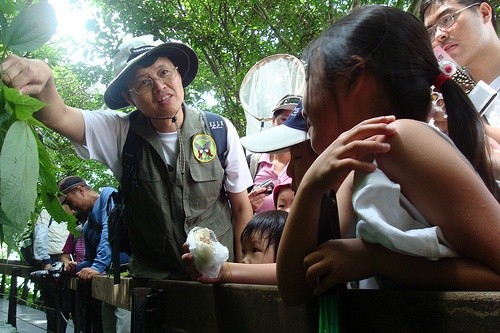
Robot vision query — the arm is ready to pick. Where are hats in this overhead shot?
[240,99,311,153]
[273,178,292,209]
[104,35,198,110]
[57,176,86,206]
[273,94,302,110]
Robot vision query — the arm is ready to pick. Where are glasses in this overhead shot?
[129,66,179,96]
[426,3,479,39]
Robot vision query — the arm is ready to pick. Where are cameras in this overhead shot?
[30,262,64,281]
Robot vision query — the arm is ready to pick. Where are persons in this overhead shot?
[181,210,289,285]
[0,39,253,333]
[420,0,500,192]
[276,6,500,306]
[32,204,86,333]
[239,93,350,241]
[57,176,129,333]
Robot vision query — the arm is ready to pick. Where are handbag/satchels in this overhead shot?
[20,243,33,265]
